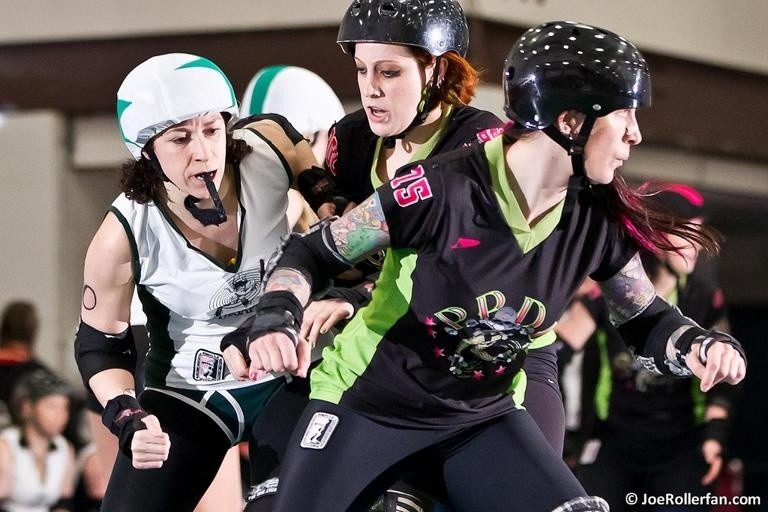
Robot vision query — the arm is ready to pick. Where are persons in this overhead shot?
[54,2,750,510]
[0,366,79,510]
[0,298,51,425]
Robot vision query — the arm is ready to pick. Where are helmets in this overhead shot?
[114,51,241,163]
[335,0,470,65]
[242,64,348,143]
[11,371,73,419]
[499,19,653,132]
